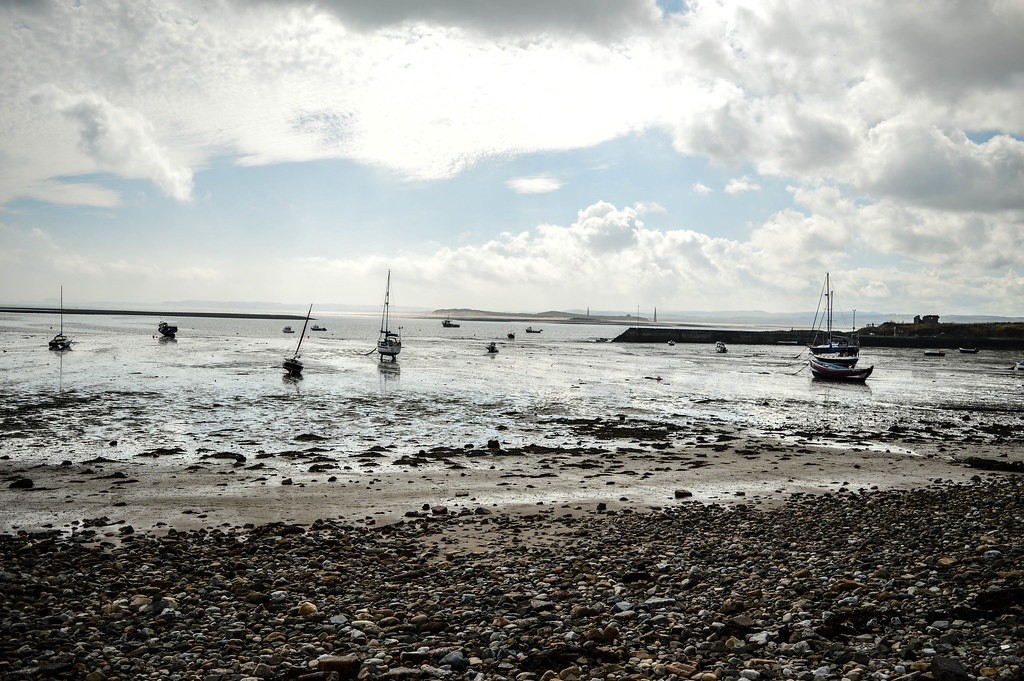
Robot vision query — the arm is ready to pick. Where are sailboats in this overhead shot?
[376,269,401,363]
[283,303,314,375]
[810,291,859,356]
[49,285,73,347]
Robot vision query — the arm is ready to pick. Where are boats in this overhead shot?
[668,340,675,345]
[715,341,726,353]
[442,319,461,327]
[924,351,945,356]
[486,342,498,352]
[311,323,327,331]
[158,320,178,339]
[809,359,874,383]
[507,332,516,338]
[959,347,977,353]
[814,353,860,368]
[526,326,543,333]
[282,325,294,333]
